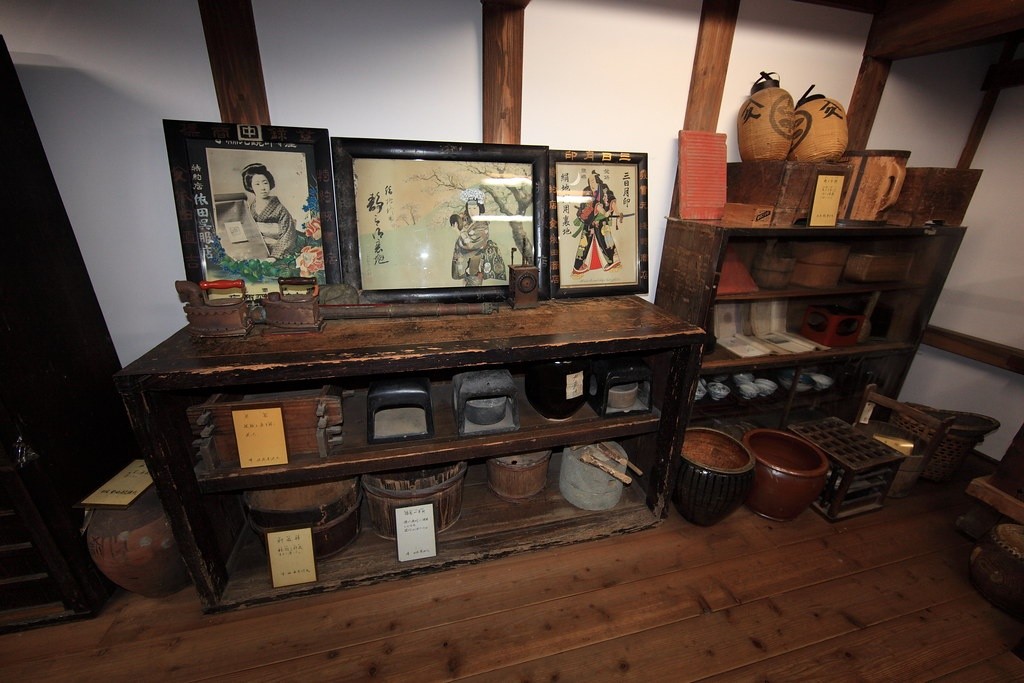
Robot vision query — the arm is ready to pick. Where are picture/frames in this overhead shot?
[162,118,342,306]
[547,150,649,299]
[329,137,550,306]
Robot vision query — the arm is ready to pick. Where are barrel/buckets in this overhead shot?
[789,242,849,288]
[241,477,361,562]
[852,384,955,498]
[485,450,552,501]
[752,257,796,290]
[839,148,911,226]
[360,461,466,539]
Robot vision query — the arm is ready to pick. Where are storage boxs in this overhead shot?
[727,162,854,225]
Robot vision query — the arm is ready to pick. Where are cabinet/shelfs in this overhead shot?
[654,216,967,432]
[111,294,709,618]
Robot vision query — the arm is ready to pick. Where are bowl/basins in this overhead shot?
[780,374,816,392]
[708,382,731,400]
[755,379,778,396]
[805,373,834,391]
[694,388,707,401]
[742,429,829,522]
[673,427,756,526]
[733,372,754,386]
[740,383,760,399]
